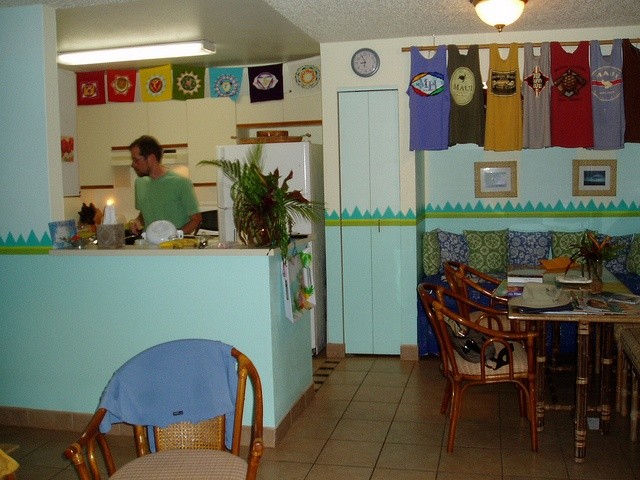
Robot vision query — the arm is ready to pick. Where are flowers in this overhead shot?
[197,140,328,260]
[564,231,624,277]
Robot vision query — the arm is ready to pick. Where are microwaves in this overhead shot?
[191,207,219,236]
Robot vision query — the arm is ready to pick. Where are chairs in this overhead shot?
[440,260,537,417]
[64,340,265,480]
[620,326,640,442]
[416,282,540,452]
[611,322,640,412]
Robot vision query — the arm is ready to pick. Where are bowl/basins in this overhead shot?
[146,220,183,245]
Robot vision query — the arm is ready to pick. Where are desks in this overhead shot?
[506,266,640,463]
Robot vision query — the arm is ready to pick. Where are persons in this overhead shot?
[128,135,202,240]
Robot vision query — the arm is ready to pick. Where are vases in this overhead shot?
[589,258,604,294]
[242,215,281,247]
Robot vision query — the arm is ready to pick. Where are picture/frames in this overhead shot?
[474,161,517,197]
[572,159,617,196]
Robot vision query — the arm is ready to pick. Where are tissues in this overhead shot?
[97,204,126,249]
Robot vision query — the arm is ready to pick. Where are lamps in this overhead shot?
[469,0,528,33]
[54,41,215,66]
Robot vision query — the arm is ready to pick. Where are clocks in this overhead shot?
[351,48,379,77]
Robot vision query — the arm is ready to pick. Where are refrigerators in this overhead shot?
[215,140,325,356]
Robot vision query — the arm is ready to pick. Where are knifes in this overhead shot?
[78,201,96,224]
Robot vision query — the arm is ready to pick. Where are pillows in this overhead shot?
[583,229,636,274]
[551,231,599,265]
[626,233,640,277]
[506,230,554,267]
[421,228,440,276]
[436,231,468,273]
[464,228,508,271]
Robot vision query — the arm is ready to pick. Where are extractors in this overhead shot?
[110,148,188,166]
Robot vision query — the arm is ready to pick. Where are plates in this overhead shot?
[229,133,312,144]
[256,130,289,136]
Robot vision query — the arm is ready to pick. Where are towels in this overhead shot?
[95,340,238,451]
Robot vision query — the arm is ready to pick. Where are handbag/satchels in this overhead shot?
[443,315,496,362]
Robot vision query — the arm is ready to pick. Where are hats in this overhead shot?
[507,282,573,310]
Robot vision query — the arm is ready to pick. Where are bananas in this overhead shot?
[177,239,199,248]
[160,240,183,249]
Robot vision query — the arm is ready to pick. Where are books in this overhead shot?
[507,273,543,283]
